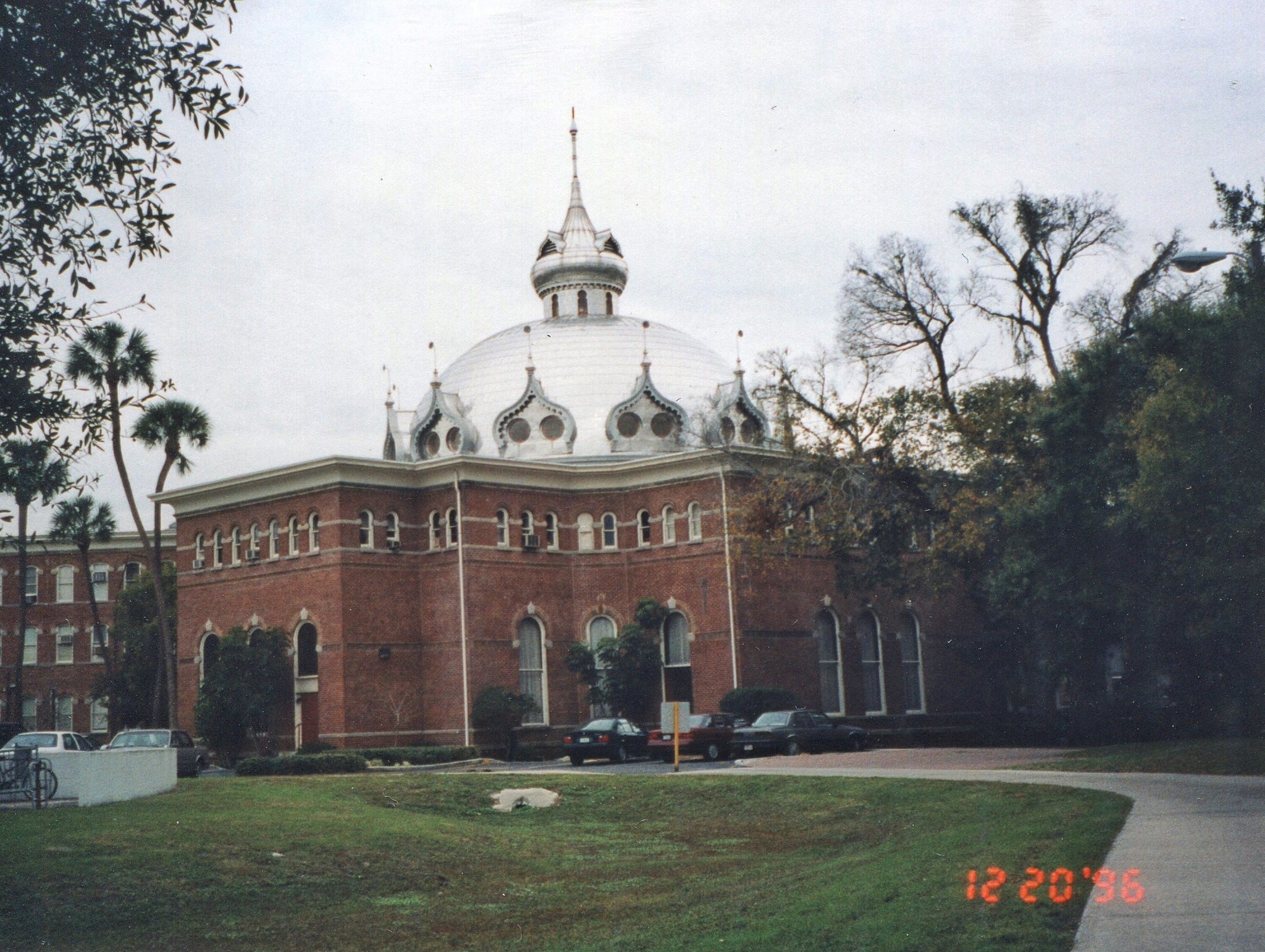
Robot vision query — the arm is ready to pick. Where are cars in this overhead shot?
[564,709,869,766]
[100,729,210,778]
[0,732,99,756]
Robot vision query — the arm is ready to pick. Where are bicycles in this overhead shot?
[0,755,56,802]
